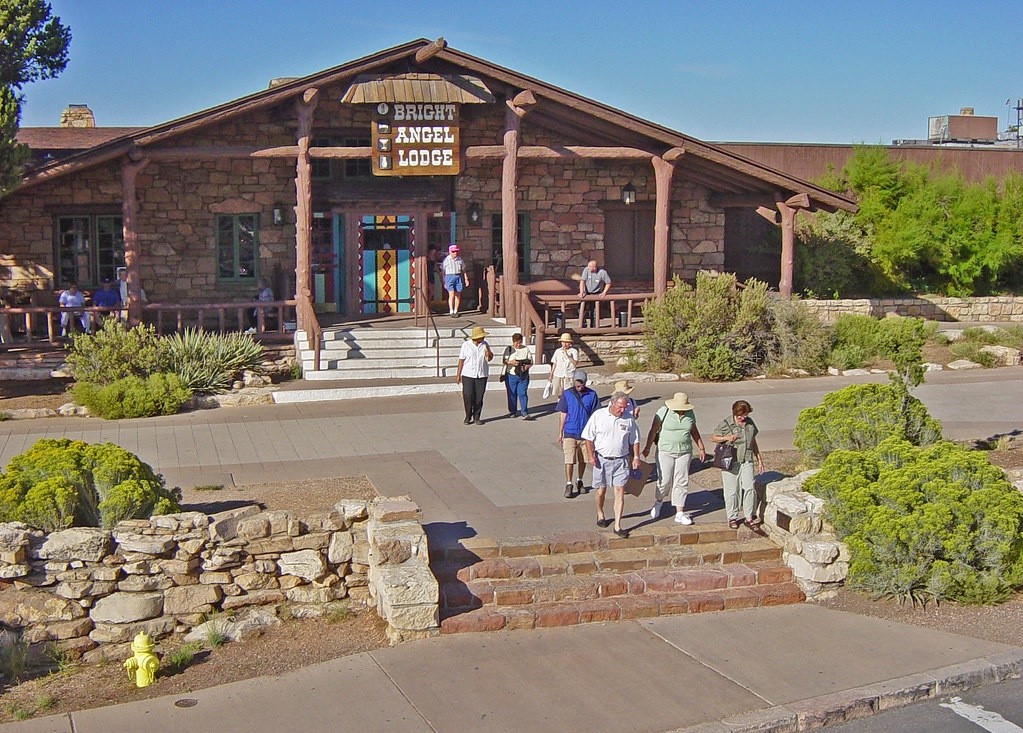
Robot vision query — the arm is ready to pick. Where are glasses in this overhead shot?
[451,251,458,253]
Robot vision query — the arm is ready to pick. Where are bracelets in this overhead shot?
[634,456,640,459]
[757,458,763,461]
[567,356,570,358]
[721,437,724,441]
[700,449,705,450]
[645,447,650,450]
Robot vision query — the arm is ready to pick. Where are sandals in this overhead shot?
[729,520,738,529]
[743,518,760,531]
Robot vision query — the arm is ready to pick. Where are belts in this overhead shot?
[599,454,624,461]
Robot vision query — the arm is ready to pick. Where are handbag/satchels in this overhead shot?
[713,419,736,472]
[500,373,507,382]
[630,398,639,419]
[653,408,670,445]
[542,381,553,399]
[624,452,654,497]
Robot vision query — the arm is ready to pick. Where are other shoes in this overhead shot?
[576,481,587,493]
[596,515,606,528]
[613,527,628,538]
[564,484,573,498]
[451,312,458,317]
[464,412,532,425]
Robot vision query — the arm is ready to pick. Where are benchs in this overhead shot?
[602,280,679,313]
[30,290,109,330]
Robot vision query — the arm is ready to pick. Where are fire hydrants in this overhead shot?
[123,631,159,687]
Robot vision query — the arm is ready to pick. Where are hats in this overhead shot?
[470,327,489,339]
[611,380,635,396]
[102,277,111,283]
[665,392,695,411]
[449,245,459,253]
[574,371,587,382]
[558,333,574,342]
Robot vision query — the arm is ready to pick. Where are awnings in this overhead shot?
[339,73,496,105]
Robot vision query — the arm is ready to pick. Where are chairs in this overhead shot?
[263,274,315,328]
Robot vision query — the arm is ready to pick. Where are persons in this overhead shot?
[503,333,534,420]
[555,369,602,498]
[578,260,611,328]
[611,381,641,494]
[440,245,469,318]
[427,244,442,313]
[58,284,92,337]
[643,392,706,525]
[709,400,764,532]
[92,268,147,334]
[244,279,275,335]
[457,326,493,425]
[581,391,641,538]
[548,333,579,403]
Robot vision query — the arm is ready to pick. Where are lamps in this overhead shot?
[468,202,482,227]
[272,200,284,227]
[623,181,636,205]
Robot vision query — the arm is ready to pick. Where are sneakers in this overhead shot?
[651,500,663,518]
[675,512,693,525]
